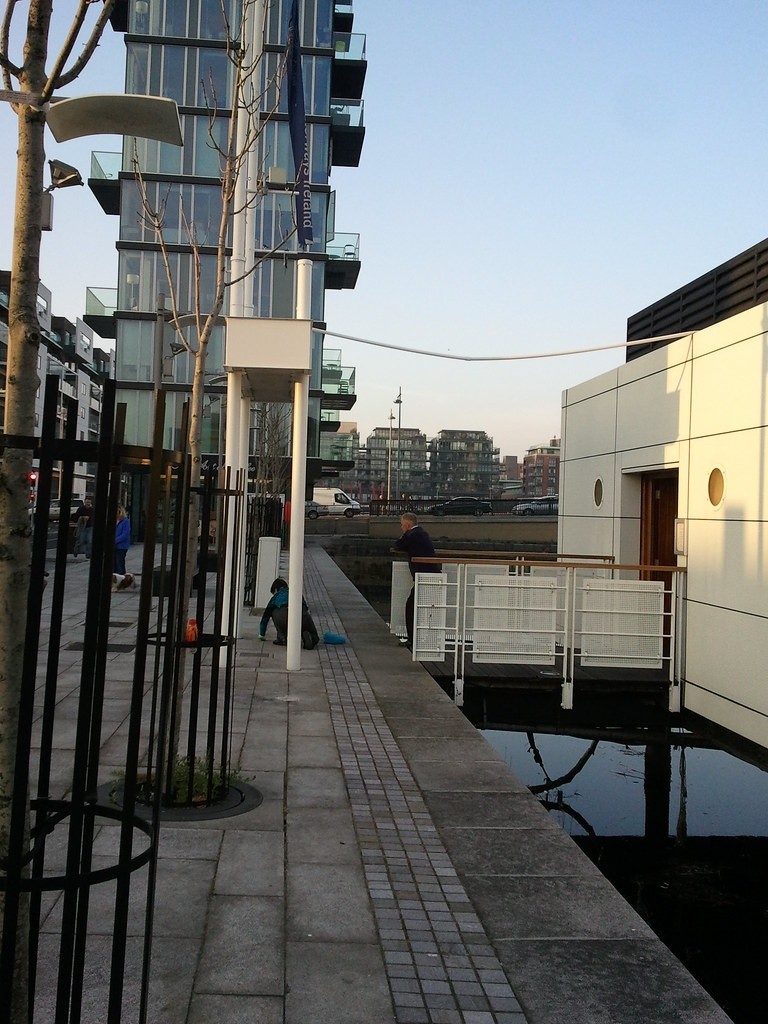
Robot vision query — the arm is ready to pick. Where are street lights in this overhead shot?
[393,386,402,500]
[386,409,396,516]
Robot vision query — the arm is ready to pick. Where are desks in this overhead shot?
[324,411,335,421]
[327,364,338,370]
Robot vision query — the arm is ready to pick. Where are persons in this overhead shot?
[260,578,308,645]
[391,512,441,647]
[73,497,93,558]
[114,504,131,575]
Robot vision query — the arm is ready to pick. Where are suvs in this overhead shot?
[27,498,86,521]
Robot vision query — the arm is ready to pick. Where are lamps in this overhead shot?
[127,274,139,310]
[135,1,148,34]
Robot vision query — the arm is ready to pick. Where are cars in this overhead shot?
[305,500,329,520]
[426,496,493,517]
[510,495,559,517]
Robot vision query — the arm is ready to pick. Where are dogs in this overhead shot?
[113,573,140,592]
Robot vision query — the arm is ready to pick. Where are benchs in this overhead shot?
[151,565,172,597]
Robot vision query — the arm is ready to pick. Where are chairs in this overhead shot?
[338,381,349,394]
[343,244,356,260]
[334,40,346,59]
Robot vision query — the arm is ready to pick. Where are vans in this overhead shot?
[312,487,363,518]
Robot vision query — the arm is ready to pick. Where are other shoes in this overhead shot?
[273,639,286,646]
[302,630,313,651]
[400,641,411,647]
